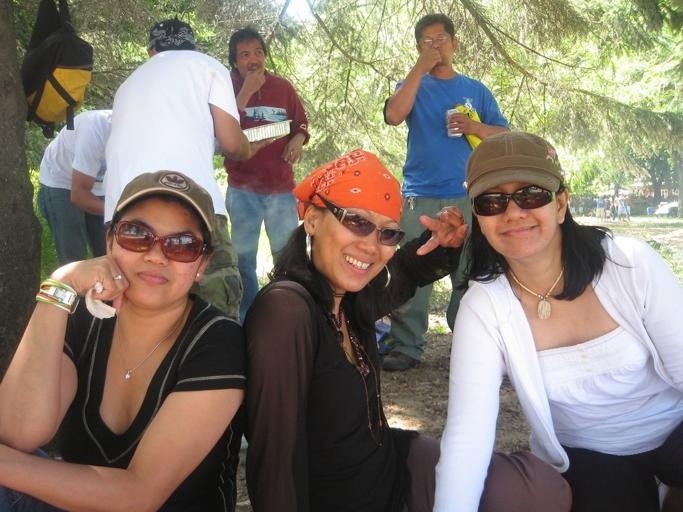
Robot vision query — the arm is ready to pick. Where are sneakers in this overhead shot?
[383,348,421,371]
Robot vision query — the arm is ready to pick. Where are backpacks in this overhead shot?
[22,2,96,137]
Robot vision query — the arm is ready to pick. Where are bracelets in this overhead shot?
[35,278,80,314]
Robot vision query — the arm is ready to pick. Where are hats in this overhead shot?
[113,168,216,235]
[467,130,563,198]
[149,18,196,51]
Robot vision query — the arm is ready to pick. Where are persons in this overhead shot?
[229,27,310,326]
[37,110,113,266]
[246,150,571,511]
[433,132,683,512]
[0,171,246,511]
[383,13,510,369]
[566,191,631,221]
[104,22,276,320]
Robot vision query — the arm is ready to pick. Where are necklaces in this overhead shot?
[510,263,567,321]
[115,303,184,380]
[322,302,371,376]
[328,306,344,342]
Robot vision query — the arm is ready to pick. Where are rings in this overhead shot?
[113,274,123,281]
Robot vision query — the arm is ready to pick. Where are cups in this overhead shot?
[447,109,464,137]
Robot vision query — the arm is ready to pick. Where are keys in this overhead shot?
[406,197,417,213]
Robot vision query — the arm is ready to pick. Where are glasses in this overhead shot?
[418,33,453,47]
[313,190,405,250]
[471,184,567,219]
[110,220,206,264]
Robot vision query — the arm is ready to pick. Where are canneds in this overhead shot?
[445,107,463,136]
